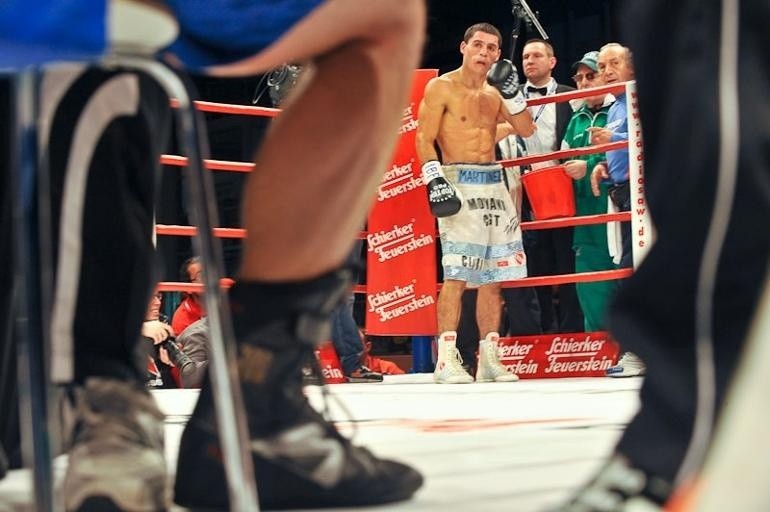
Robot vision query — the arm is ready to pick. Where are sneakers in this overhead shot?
[51,372,169,512]
[346,364,383,383]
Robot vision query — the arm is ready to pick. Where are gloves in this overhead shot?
[487,60,528,116]
[421,159,463,218]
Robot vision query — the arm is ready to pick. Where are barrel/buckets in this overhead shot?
[519,164,576,220]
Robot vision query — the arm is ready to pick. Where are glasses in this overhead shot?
[571,73,598,82]
[153,292,164,301]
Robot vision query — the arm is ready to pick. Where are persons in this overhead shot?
[1,0,429,509]
[492,132,534,226]
[540,1,770,508]
[588,40,649,378]
[516,36,582,333]
[0,66,174,509]
[410,19,536,386]
[558,48,619,334]
[127,255,408,384]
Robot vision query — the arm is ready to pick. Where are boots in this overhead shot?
[171,264,425,512]
[432,330,475,384]
[474,331,520,383]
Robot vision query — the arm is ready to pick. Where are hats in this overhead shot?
[571,51,600,73]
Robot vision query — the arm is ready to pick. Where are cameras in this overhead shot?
[148,322,197,378]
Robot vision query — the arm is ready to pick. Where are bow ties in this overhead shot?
[527,86,547,96]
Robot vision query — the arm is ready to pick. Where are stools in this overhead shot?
[0,0,263,511]
